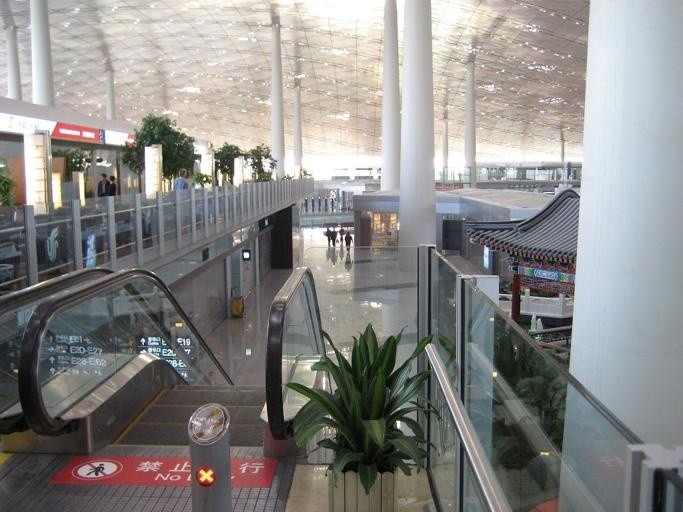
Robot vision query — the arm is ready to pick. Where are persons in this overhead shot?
[345,253,352,271]
[344,232,353,248]
[326,246,330,261]
[173,169,188,191]
[330,229,337,246]
[338,247,343,260]
[339,227,344,241]
[326,229,330,243]
[107,175,115,195]
[331,247,336,264]
[96,173,109,196]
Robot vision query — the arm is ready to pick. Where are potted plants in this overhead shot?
[284,322,442,511]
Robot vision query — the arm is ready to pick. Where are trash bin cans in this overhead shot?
[232,296,245,318]
